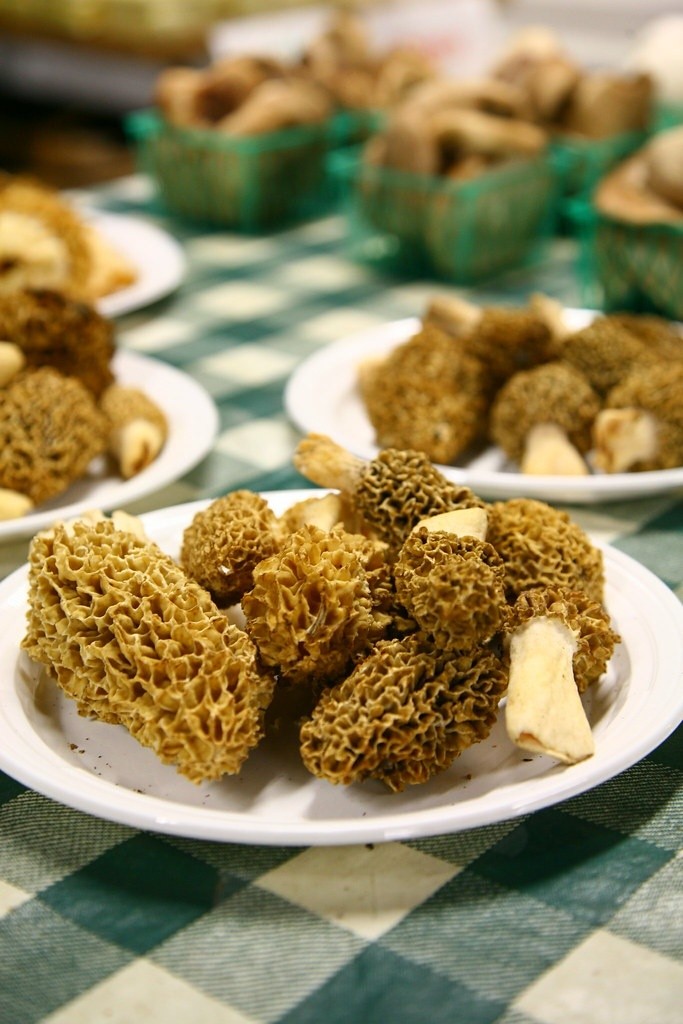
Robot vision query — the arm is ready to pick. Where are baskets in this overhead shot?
[123,105,682,319]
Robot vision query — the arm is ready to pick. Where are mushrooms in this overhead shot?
[0,185,683,794]
[157,21,683,317]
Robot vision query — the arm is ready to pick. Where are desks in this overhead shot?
[0,169,683,1024]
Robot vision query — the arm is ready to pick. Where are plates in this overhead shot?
[0,350,220,549]
[1,485,683,846]
[79,207,188,321]
[281,305,683,506]
